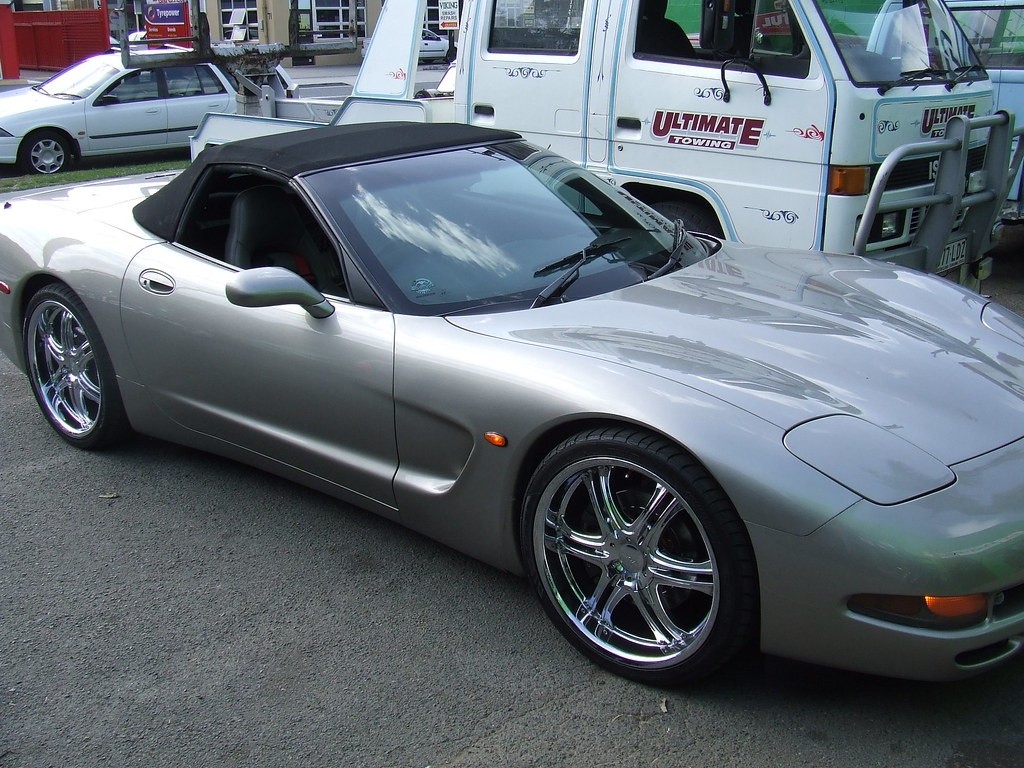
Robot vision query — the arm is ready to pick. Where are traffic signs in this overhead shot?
[439,0,459,30]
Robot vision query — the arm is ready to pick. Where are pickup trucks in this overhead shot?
[361,27,458,65]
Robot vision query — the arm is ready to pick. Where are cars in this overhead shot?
[0,45,258,176]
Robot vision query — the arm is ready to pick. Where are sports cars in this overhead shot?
[0,118,1024,693]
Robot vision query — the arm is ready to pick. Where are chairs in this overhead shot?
[227,183,352,298]
[636,0,699,58]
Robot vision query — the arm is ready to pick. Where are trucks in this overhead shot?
[188,0,1024,345]
